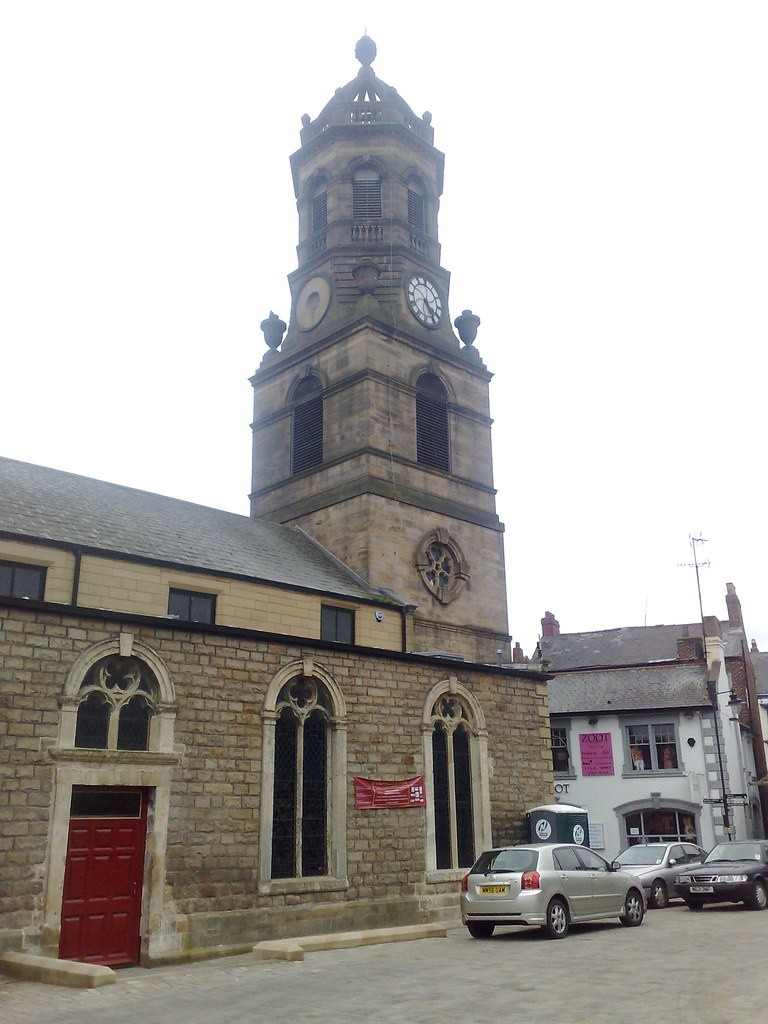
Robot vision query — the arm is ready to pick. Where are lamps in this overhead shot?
[589,717,599,727]
[683,712,693,720]
[695,709,701,716]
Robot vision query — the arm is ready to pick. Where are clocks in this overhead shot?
[407,271,446,329]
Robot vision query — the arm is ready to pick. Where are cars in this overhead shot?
[615,842,709,910]
[674,839,768,911]
[460,843,649,940]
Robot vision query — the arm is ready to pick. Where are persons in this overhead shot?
[664,745,674,768]
[631,746,645,770]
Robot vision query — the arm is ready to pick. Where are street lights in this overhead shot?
[712,690,742,842]
